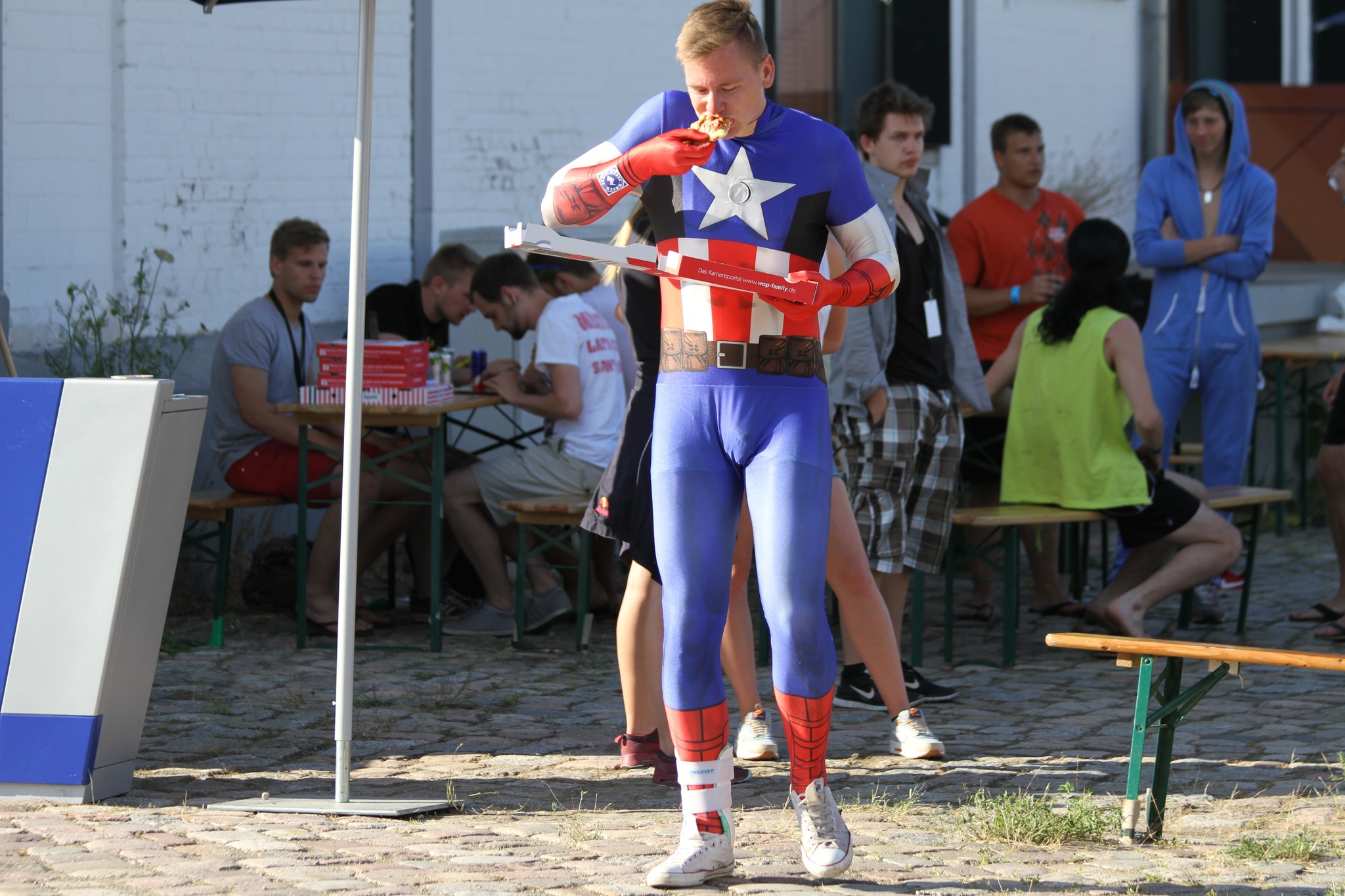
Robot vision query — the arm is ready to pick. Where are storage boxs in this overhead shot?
[299,338,454,406]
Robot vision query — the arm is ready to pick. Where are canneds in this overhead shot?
[470,349,488,392]
[1047,279,1063,304]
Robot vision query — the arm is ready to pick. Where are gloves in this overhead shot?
[553,128,714,226]
[759,257,895,321]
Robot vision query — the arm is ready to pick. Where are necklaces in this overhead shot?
[1199,176,1222,204]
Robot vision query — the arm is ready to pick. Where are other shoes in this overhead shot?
[407,594,471,622]
[1208,568,1244,590]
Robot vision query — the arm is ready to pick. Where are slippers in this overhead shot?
[1027,601,1085,617]
[1289,601,1345,621]
[357,605,391,626]
[1315,621,1345,641]
[954,603,994,619]
[305,620,375,638]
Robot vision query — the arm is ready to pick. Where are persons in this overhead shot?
[344,0,1345,887]
[211,218,430,637]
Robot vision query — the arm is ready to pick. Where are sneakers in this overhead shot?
[886,707,946,759]
[442,600,515,636]
[520,587,571,630]
[733,706,779,760]
[651,748,751,786]
[647,814,735,886]
[615,729,660,769]
[832,661,959,709]
[789,777,853,877]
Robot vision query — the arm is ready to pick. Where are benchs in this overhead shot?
[908,441,1345,850]
[188,486,594,651]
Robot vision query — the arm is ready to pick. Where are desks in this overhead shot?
[1259,331,1345,538]
[261,384,509,653]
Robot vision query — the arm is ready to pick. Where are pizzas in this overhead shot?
[683,116,733,145]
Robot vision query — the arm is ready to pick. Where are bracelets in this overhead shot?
[1146,447,1164,454]
[1008,285,1022,306]
